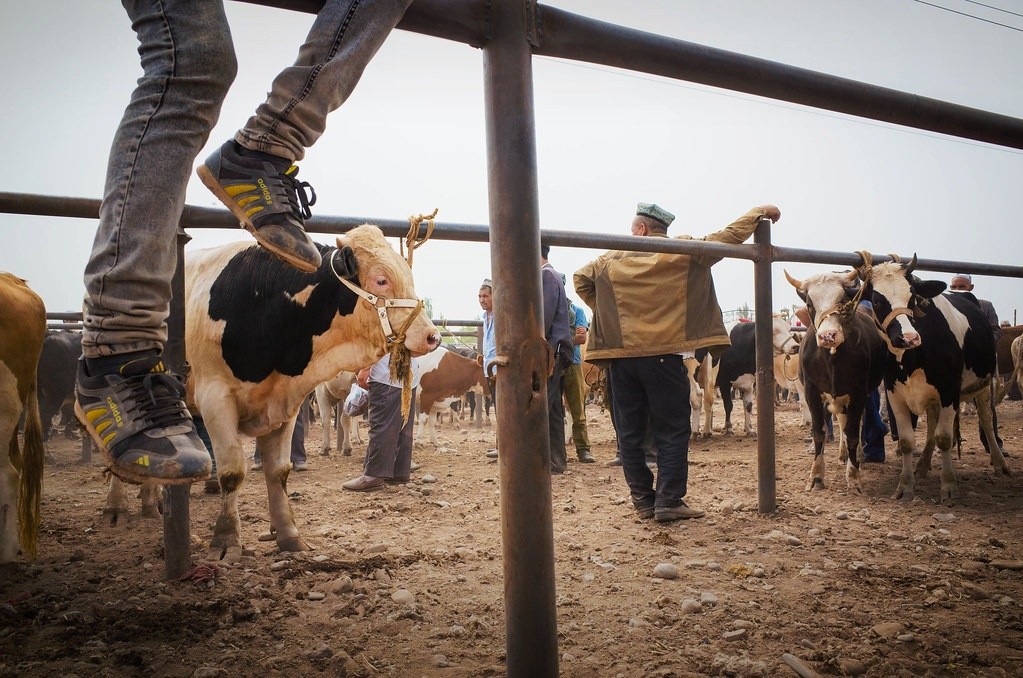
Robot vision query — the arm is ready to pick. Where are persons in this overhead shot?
[342,352,419,491]
[478,278,498,458]
[251,404,306,470]
[836,269,889,463]
[71,0,414,486]
[950,273,1008,458]
[886,398,921,456]
[574,201,780,522]
[541,243,593,472]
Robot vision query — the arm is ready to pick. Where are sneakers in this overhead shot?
[195,138,321,272]
[73,353,212,488]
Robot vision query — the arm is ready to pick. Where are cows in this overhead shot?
[415,345,490,451]
[0,270,47,565]
[684,310,812,442]
[784,253,1023,502]
[102,224,441,569]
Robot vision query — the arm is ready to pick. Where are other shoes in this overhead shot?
[654,499,706,522]
[486,451,499,457]
[910,448,922,455]
[251,462,263,470]
[605,457,623,467]
[638,504,655,518]
[578,449,596,462]
[985,447,1008,457]
[292,460,307,470]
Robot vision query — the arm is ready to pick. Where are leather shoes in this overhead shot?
[342,475,384,491]
[381,477,410,484]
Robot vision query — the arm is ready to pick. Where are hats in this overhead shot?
[558,272,566,284]
[637,202,675,226]
[481,278,492,288]
